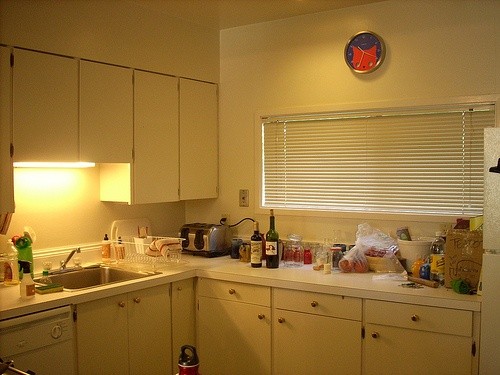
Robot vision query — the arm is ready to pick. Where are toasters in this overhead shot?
[177,222,234,254]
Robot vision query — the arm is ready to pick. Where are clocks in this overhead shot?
[343,31,386,74]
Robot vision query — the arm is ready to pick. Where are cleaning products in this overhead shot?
[114,236,125,261]
[17,259,35,298]
[101,233,111,261]
[3,239,19,287]
[16,236,34,281]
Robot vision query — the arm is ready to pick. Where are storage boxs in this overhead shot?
[444,229,483,288]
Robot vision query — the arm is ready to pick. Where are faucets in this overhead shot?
[60,247,81,272]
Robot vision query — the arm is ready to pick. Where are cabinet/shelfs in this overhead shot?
[12,48,133,168]
[95,62,219,204]
[73,279,480,375]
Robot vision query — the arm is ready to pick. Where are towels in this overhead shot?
[144,237,182,257]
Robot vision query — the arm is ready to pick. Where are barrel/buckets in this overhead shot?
[397,236,435,273]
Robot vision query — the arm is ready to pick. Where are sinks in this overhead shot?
[31,263,163,293]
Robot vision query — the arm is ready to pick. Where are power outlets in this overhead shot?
[239,190,249,207]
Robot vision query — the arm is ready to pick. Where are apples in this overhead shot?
[339,259,367,273]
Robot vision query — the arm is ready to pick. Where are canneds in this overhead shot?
[328,247,343,270]
[230,238,251,262]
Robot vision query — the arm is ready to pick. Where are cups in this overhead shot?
[239,242,250,262]
[231,238,242,259]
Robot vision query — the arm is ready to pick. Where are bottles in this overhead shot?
[428,231,446,285]
[250,222,263,267]
[116,237,125,259]
[4,239,19,286]
[264,210,280,269]
[175,345,200,375]
[102,234,111,261]
[283,233,356,275]
[395,226,411,241]
[38,271,52,284]
[15,237,35,281]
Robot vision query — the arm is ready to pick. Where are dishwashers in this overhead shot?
[0,305,75,375]
